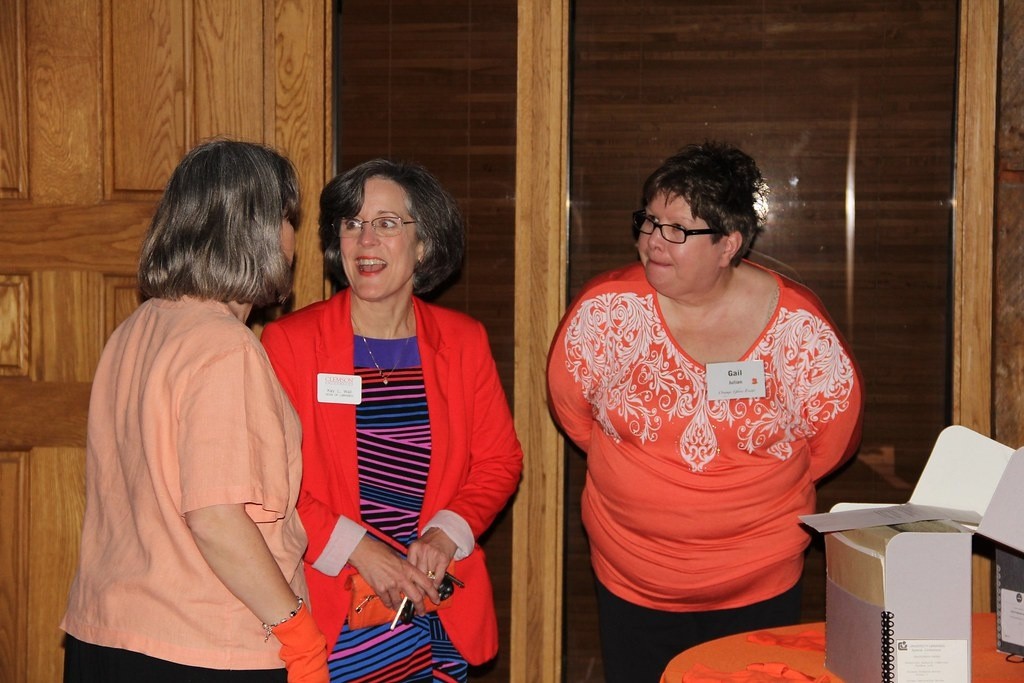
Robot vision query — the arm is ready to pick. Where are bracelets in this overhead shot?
[262,597,302,642]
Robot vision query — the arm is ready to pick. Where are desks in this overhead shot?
[659,613,1024,683]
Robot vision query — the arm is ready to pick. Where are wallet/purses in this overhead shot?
[344,571,453,630]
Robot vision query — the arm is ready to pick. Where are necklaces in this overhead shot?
[351,303,414,385]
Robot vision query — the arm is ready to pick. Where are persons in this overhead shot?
[259,161,524,683]
[548,143,861,683]
[58,141,328,683]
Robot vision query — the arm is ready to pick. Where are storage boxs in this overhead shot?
[824,424,1024,683]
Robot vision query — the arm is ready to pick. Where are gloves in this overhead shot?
[271,601,331,683]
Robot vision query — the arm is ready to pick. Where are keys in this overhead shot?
[389,572,466,631]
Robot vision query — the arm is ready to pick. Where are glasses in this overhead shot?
[331,215,418,238]
[633,209,725,244]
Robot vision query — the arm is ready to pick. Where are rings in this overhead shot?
[427,571,435,577]
